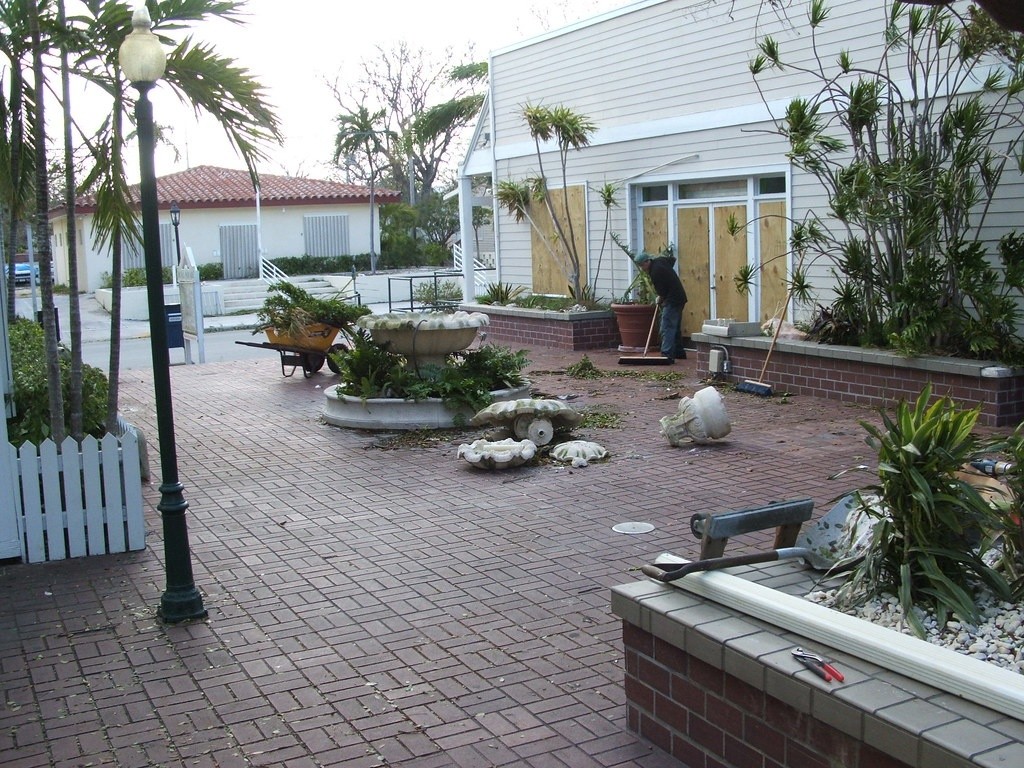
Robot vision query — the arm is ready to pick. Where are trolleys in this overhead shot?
[235,320,348,378]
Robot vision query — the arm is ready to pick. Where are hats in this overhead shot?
[634,253,654,262]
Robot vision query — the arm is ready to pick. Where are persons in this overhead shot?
[635,253,687,362]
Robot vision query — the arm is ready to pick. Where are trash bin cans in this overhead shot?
[37,307,60,343]
[166,303,184,347]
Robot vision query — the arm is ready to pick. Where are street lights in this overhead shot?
[119,4,210,622]
[169,201,181,266]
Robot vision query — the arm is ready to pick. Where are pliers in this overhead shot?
[791,647,845,682]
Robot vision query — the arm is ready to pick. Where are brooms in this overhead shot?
[734,248,807,397]
[618,304,672,366]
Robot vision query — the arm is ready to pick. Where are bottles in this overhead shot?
[969,458,1014,476]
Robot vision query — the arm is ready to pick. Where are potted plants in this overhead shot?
[610,232,676,349]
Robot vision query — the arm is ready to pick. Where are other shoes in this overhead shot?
[661,352,674,363]
[673,351,686,359]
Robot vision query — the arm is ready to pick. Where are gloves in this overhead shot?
[655,296,662,304]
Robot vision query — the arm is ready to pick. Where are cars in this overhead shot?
[14,264,30,282]
